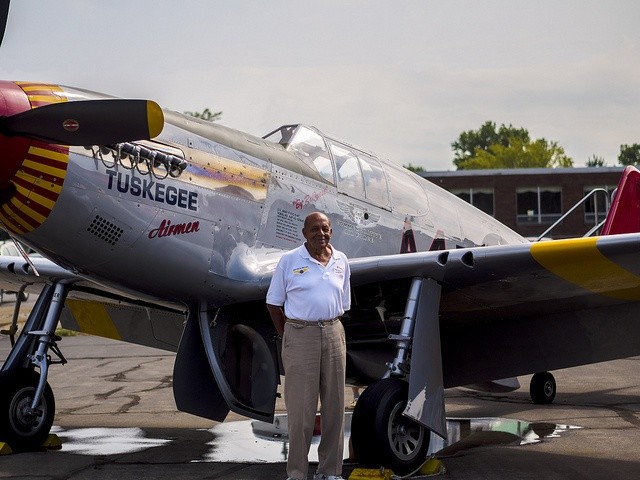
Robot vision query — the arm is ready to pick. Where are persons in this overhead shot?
[265,212,353,480]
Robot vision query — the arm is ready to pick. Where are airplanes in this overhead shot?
[0,81,640,476]
